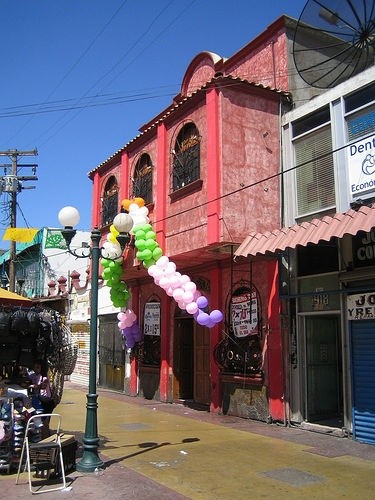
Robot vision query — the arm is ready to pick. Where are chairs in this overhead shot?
[15,413,66,494]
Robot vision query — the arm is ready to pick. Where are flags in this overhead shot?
[3,227,39,243]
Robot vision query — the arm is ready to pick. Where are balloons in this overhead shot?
[102,196,223,354]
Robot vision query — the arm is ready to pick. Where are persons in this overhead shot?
[0,385,33,425]
[28,359,52,411]
[13,398,36,453]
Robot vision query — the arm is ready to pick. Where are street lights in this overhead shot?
[58,205,135,473]
